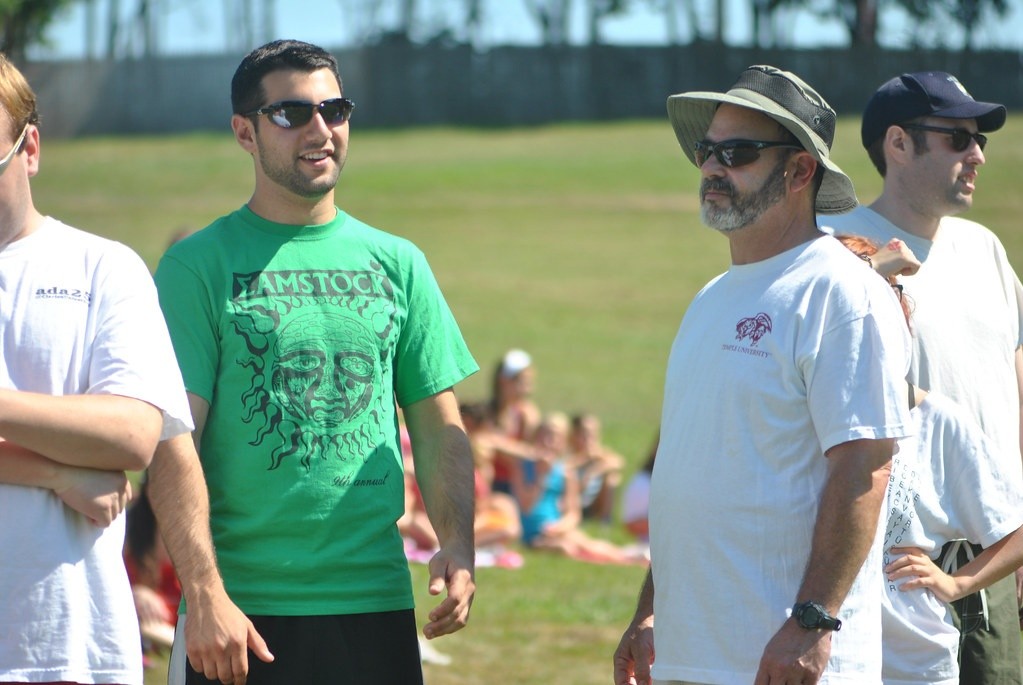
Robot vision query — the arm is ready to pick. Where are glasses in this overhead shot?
[901,123,987,151]
[242,98,355,129]
[693,139,803,167]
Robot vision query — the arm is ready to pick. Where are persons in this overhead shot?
[613,64,911,685]
[833,235,1023,685]
[395,348,660,550]
[722,148,732,166]
[331,102,350,122]
[816,71,1023,685]
[273,107,290,127]
[122,479,183,668]
[0,52,196,684]
[146,40,481,685]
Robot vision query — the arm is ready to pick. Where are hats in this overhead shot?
[861,70,1007,147]
[667,63,859,214]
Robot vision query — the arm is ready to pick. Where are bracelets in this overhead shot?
[861,255,872,269]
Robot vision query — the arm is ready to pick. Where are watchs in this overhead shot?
[791,600,842,631]
